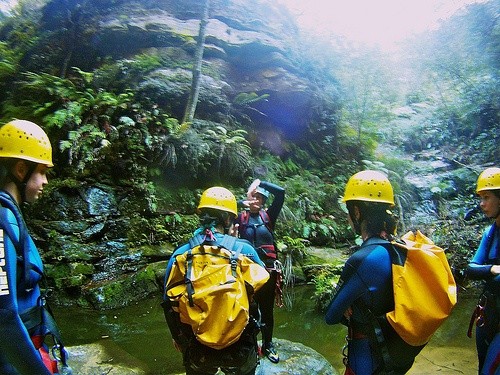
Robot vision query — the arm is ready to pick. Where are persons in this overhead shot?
[0,119,73,375]
[228,178,285,365]
[464,167,500,375]
[324,170,435,375]
[162,185,271,375]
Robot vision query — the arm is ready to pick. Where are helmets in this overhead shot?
[256,187,269,203]
[477,167,500,194]
[0,119,54,167]
[341,169,395,207]
[197,186,238,220]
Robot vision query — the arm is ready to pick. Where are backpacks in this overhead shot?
[357,230,458,359]
[164,235,270,361]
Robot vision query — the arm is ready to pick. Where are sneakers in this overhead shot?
[262,345,279,364]
